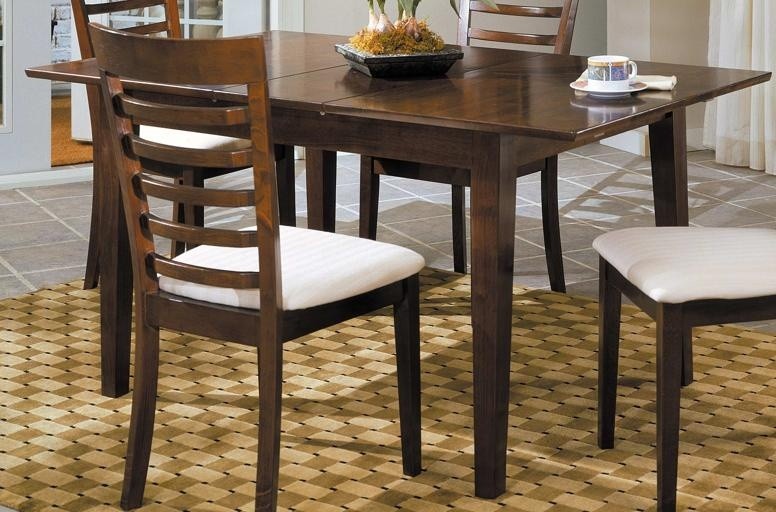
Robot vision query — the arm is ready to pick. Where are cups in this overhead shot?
[588,54,638,91]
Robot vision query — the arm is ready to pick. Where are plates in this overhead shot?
[570,96,645,110]
[569,80,646,99]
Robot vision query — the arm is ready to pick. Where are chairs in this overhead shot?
[68,1,255,293]
[87,19,428,511]
[591,223,774,511]
[357,1,579,296]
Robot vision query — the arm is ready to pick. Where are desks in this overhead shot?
[25,28,770,498]
